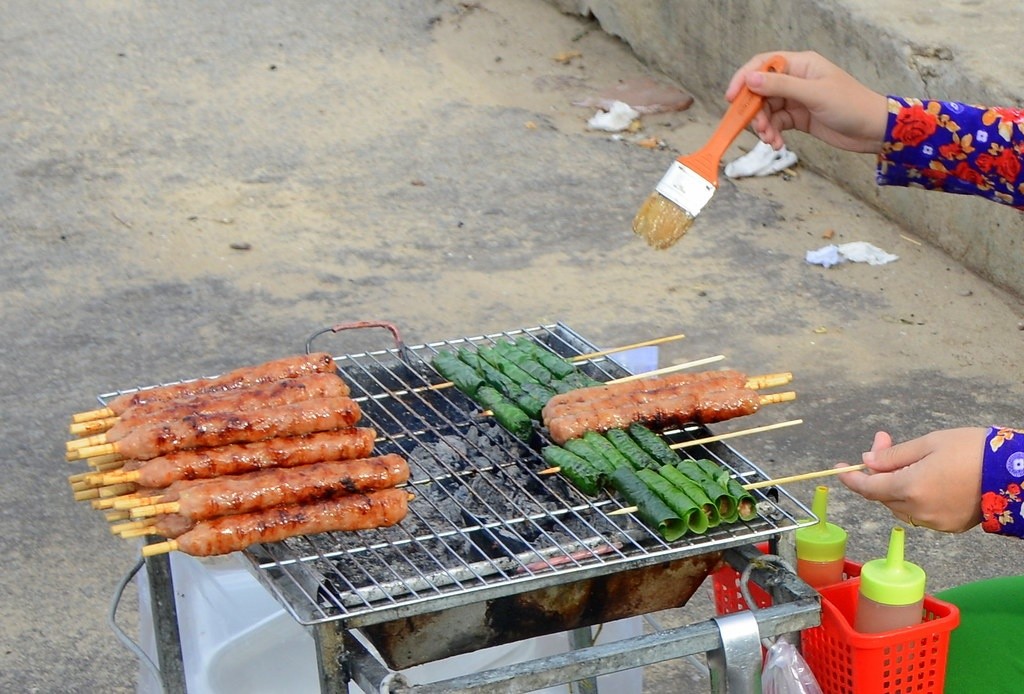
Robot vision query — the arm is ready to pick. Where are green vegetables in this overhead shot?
[609,459,759,541]
[433,336,682,491]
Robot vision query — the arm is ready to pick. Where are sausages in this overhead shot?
[105,350,412,557]
[542,369,758,432]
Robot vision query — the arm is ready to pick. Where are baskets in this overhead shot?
[708,545,960,693]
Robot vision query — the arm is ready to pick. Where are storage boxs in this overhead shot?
[714,541,961,694]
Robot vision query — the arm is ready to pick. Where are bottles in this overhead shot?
[796,485,846,591]
[852,526,926,673]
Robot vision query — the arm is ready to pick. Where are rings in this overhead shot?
[908,515,917,528]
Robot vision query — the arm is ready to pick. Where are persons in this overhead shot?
[726,46,1024,543]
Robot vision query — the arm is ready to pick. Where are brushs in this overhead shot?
[631,55,788,252]
